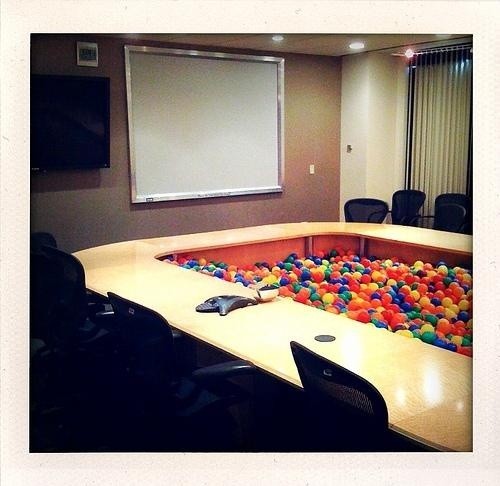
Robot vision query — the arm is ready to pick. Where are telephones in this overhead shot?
[196,296,258,315]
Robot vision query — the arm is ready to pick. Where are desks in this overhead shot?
[71,221,473,451]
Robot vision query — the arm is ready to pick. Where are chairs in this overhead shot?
[40,245,122,399]
[285,337,392,452]
[31,231,102,357]
[104,288,267,451]
[389,187,427,225]
[341,196,388,223]
[430,191,473,233]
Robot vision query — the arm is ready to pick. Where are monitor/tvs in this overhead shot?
[30,76,111,175]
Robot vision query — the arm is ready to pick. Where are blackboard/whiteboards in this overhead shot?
[123,45,284,203]
